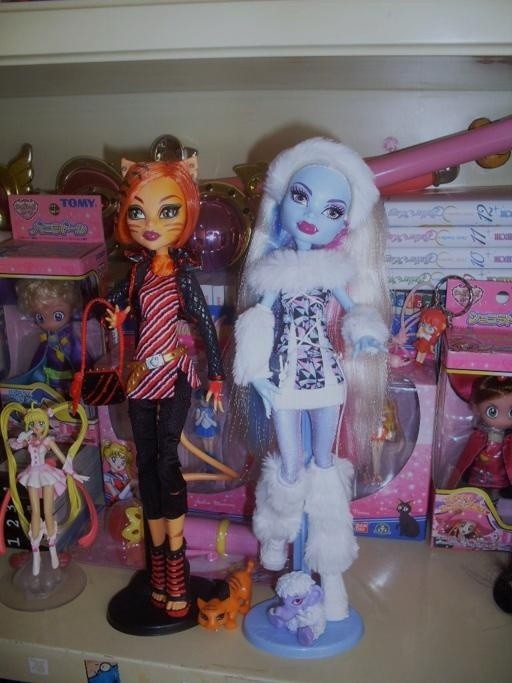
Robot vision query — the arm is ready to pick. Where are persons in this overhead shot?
[1,136,512,659]
